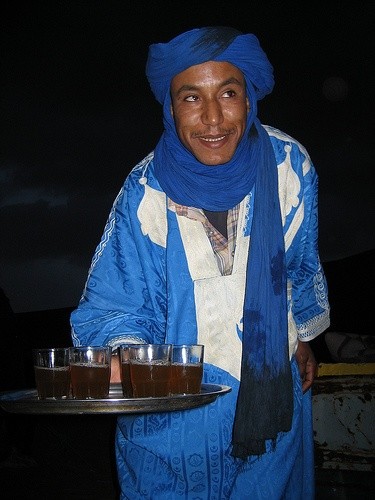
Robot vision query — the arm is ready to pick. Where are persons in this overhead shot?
[67,26,331,500]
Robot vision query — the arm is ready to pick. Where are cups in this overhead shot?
[32,348,70,400]
[170,345,204,395]
[70,347,111,400]
[119,344,170,399]
